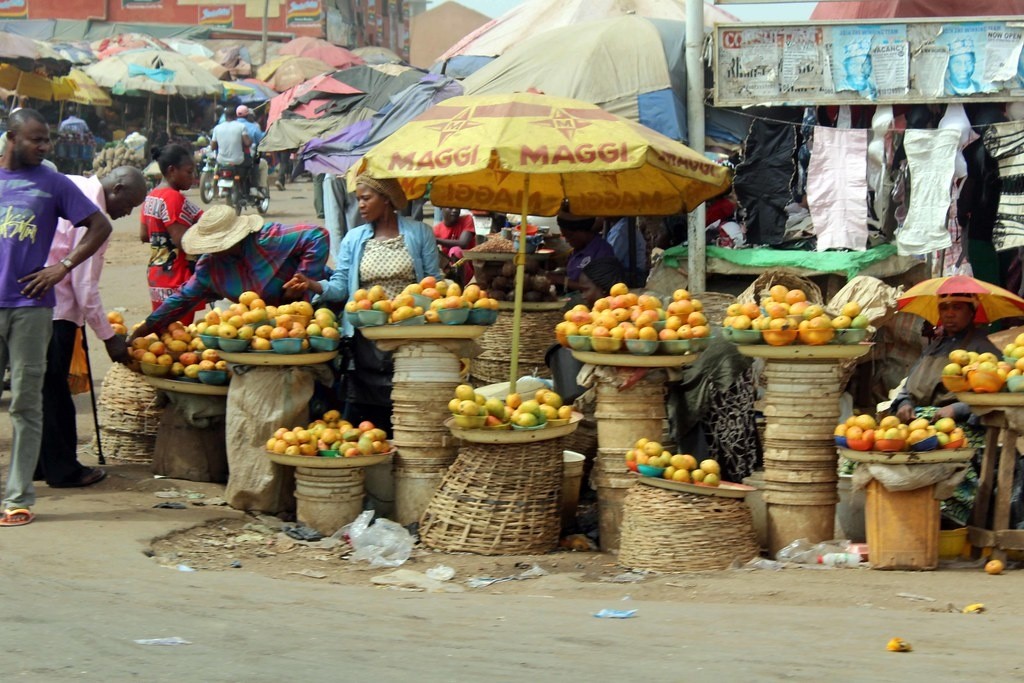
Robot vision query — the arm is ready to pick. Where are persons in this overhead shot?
[60,106,89,138]
[433,207,475,286]
[0,108,145,526]
[205,101,265,200]
[841,292,1004,528]
[141,144,208,325]
[551,256,624,402]
[534,213,647,291]
[324,173,365,264]
[283,174,442,373]
[129,204,328,342]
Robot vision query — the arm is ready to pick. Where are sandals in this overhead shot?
[50,468,106,487]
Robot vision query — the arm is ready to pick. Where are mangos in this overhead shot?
[625,437,721,485]
[722,285,869,331]
[943,333,1024,378]
[109,291,339,379]
[448,384,572,426]
[345,275,498,323]
[832,414,964,448]
[554,283,711,341]
[265,408,390,457]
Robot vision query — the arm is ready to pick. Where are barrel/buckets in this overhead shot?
[390,341,463,527]
[561,450,585,512]
[292,467,366,536]
[761,359,843,556]
[592,364,670,553]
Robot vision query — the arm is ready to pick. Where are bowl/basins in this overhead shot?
[720,308,867,345]
[938,528,968,561]
[346,292,498,327]
[501,224,550,254]
[833,434,963,452]
[940,354,1024,392]
[126,314,339,387]
[554,318,715,352]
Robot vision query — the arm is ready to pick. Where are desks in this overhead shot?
[963,411,1024,568]
[661,245,928,304]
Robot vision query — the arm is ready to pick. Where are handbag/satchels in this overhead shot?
[67,327,90,394]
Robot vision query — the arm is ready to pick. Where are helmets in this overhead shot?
[236,105,248,116]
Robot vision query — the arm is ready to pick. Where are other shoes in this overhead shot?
[275,181,285,191]
[249,186,265,198]
[259,189,269,197]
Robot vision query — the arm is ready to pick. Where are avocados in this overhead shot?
[472,259,553,302]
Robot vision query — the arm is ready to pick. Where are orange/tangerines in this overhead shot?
[984,559,1004,574]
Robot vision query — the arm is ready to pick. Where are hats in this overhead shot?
[355,176,408,211]
[558,216,596,232]
[583,257,625,294]
[181,205,264,255]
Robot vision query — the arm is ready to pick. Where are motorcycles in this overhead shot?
[199,129,271,216]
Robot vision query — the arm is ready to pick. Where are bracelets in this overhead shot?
[564,276,568,285]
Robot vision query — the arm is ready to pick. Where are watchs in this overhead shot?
[60,258,74,271]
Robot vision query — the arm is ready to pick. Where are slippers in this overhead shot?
[0,509,35,526]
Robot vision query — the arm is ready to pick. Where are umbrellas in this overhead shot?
[348,0,743,396]
[0,33,403,124]
[895,274,1024,328]
